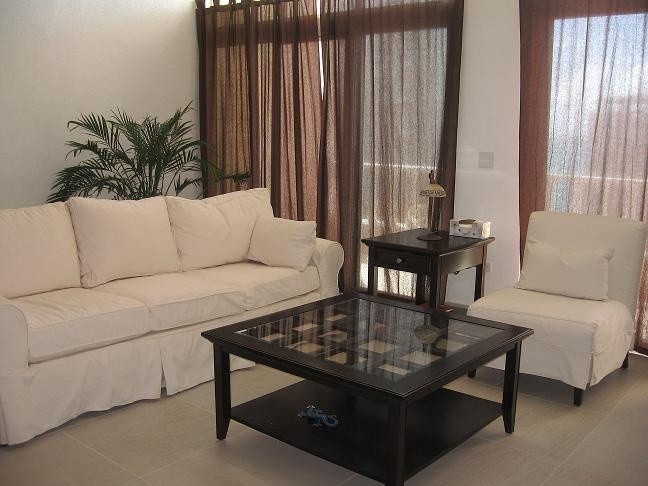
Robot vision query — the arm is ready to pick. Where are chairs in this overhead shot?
[462,210,648,407]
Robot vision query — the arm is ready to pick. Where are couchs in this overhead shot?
[0,187,344,451]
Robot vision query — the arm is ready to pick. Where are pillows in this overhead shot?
[514,231,615,305]
[247,213,318,272]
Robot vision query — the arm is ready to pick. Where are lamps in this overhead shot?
[417,169,448,241]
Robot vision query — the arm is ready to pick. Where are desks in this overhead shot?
[360,228,495,310]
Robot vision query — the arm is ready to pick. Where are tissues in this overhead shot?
[450,218,491,240]
